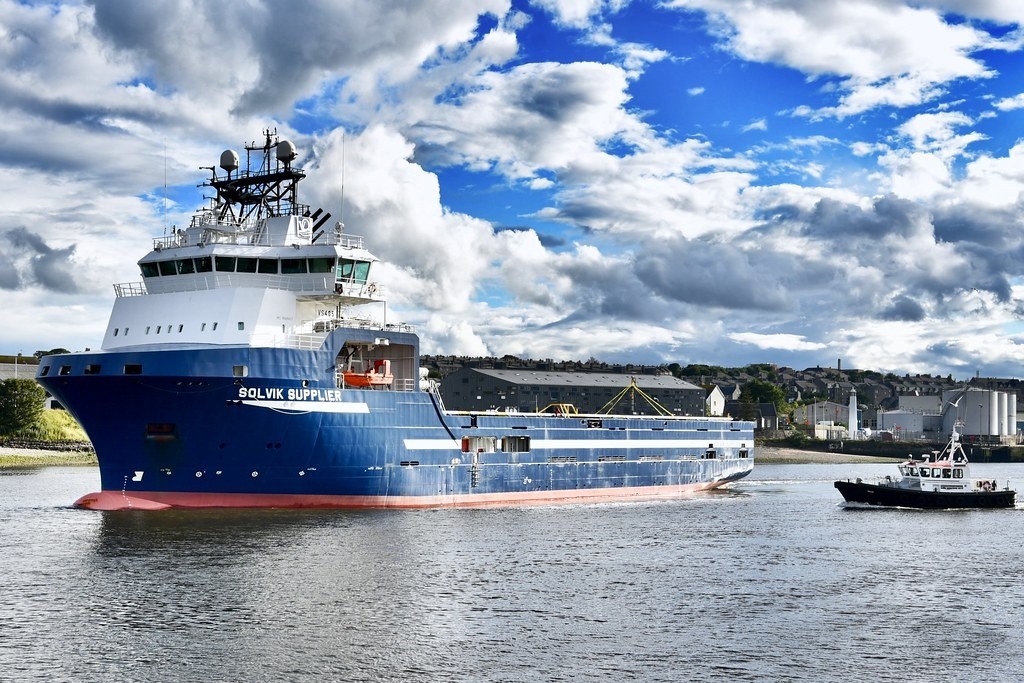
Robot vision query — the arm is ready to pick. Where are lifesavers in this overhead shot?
[982,481,991,491]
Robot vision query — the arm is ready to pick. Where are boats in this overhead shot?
[35,128,757,511]
[834,424,1017,510]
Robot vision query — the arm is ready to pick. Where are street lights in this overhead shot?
[978,404,983,440]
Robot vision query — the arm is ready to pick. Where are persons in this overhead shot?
[906,467,997,493]
